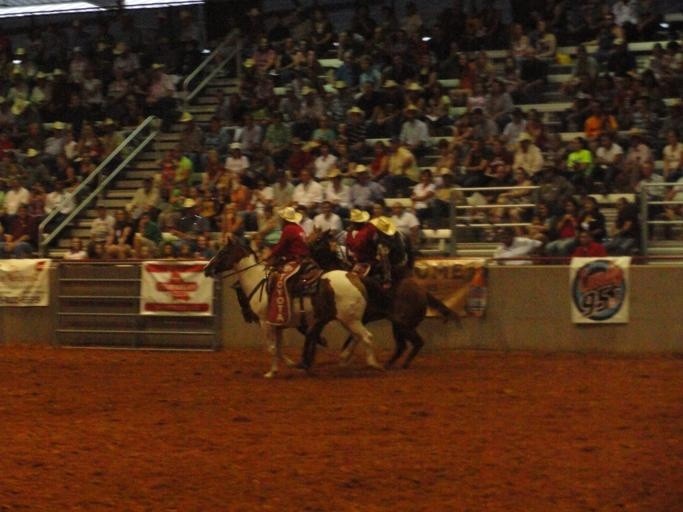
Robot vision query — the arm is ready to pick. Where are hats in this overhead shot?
[389,136,400,144]
[181,198,196,208]
[103,118,115,126]
[229,143,242,151]
[178,112,193,123]
[272,164,454,236]
[516,131,533,143]
[113,43,127,55]
[285,78,426,118]
[8,46,84,160]
[150,63,165,72]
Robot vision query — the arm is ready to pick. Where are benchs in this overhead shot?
[44,40,682,255]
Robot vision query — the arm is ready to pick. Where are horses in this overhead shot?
[203,234,385,380]
[304,224,464,370]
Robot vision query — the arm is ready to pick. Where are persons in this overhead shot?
[260,210,313,324]
[2,2,683,285]
